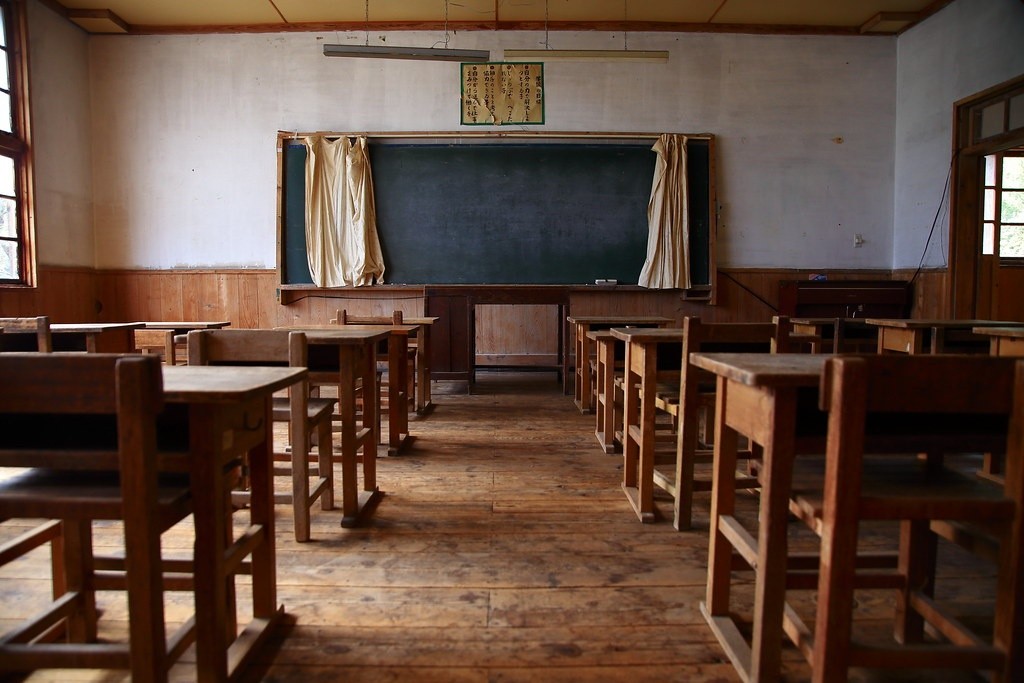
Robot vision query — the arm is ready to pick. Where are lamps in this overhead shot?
[504,0,670,64]
[323,0,490,62]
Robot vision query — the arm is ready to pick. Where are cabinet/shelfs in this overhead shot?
[423,285,571,396]
[777,278,912,319]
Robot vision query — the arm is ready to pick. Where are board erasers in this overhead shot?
[595,280,617,285]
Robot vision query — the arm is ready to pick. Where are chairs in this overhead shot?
[336,308,417,412]
[653,317,790,531]
[187,329,339,542]
[834,318,879,353]
[783,352,1024,682]
[931,326,990,353]
[0,352,237,683]
[0,316,53,352]
[134,328,187,366]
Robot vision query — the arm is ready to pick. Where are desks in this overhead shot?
[0,317,440,683]
[567,317,1024,683]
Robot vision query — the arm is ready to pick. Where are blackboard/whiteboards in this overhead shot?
[276,129,717,290]
